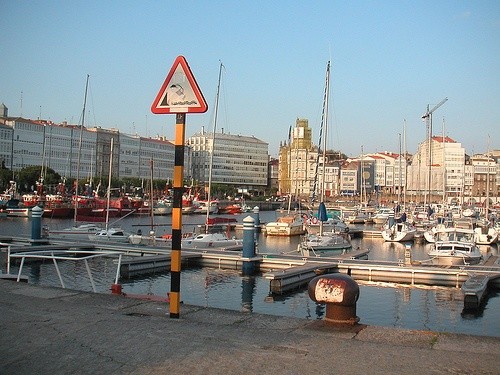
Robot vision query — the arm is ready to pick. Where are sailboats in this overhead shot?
[1,59,500,266]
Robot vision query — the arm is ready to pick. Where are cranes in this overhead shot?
[423,96,448,139]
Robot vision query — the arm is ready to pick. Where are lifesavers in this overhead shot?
[292,213,309,225]
[311,217,318,224]
